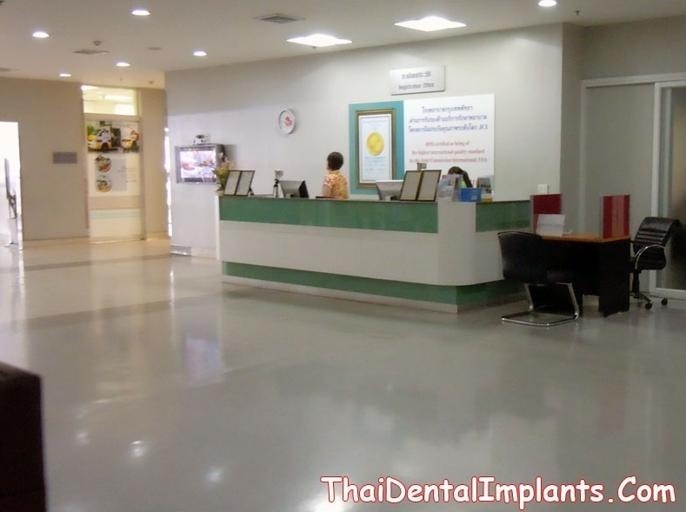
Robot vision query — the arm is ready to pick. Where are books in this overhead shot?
[436,174,461,201]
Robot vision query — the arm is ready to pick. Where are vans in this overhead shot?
[87,124,139,152]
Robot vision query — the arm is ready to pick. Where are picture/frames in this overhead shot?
[356,108,395,190]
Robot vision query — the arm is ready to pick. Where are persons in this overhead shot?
[101,128,111,153]
[128,130,139,149]
[447,166,473,188]
[323,152,349,199]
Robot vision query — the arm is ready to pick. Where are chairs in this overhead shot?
[497,229,580,328]
[630,216,676,310]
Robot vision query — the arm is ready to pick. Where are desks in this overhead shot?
[526,235,633,318]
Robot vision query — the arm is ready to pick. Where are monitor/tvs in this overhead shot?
[278,180,308,198]
[176,145,224,183]
[376,180,404,200]
